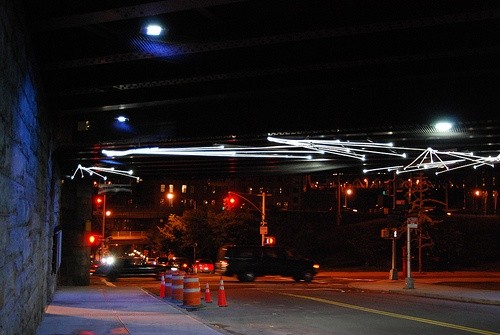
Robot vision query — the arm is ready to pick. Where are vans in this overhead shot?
[219,244,321,282]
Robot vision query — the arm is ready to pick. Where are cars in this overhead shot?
[328,203,358,217]
[416,206,460,224]
[130,257,215,274]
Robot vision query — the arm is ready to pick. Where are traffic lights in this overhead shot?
[227,194,237,208]
[87,233,97,245]
[266,237,275,244]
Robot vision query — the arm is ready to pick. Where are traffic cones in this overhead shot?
[217,277,228,307]
[204,283,214,304]
[158,272,166,298]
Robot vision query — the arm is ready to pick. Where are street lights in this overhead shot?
[345,190,352,206]
[166,192,197,259]
